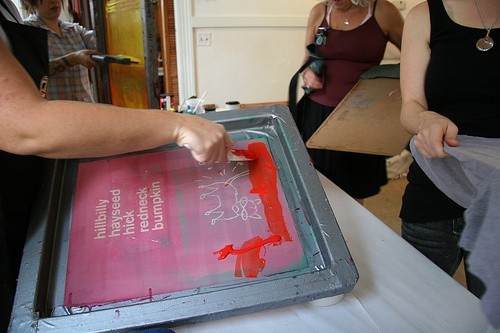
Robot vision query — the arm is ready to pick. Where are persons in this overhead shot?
[399,0,500,300]
[0,0,232,333]
[295,0,405,206]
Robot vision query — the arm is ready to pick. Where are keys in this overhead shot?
[315,26,328,46]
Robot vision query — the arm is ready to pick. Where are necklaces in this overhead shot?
[474,0,500,51]
[343,15,353,25]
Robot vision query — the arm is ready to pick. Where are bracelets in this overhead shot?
[302,69,308,81]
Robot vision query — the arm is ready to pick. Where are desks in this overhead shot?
[168,168,500,333]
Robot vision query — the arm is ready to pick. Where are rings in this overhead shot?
[306,84,309,88]
